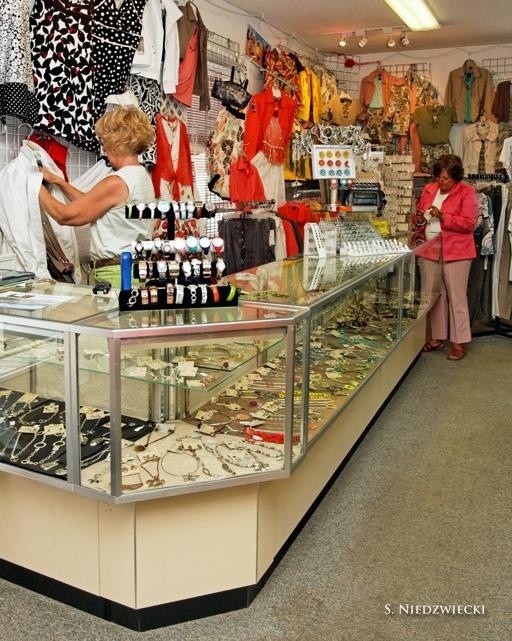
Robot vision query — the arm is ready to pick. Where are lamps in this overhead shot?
[338,37,346,48]
[387,33,395,48]
[358,35,367,48]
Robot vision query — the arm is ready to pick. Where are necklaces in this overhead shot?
[52,444,111,476]
[214,442,272,470]
[176,435,203,451]
[90,429,111,444]
[139,453,165,488]
[0,423,16,459]
[21,429,66,469]
[10,423,41,464]
[195,325,388,446]
[148,260,154,280]
[134,424,175,453]
[187,441,236,477]
[239,440,283,459]
[4,394,31,421]
[18,403,59,426]
[160,448,201,482]
[80,416,101,445]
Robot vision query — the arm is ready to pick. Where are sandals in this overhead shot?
[421,342,445,352]
[448,348,467,361]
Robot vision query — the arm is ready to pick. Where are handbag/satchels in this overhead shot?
[211,65,253,109]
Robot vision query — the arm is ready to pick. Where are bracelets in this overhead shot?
[136,199,147,221]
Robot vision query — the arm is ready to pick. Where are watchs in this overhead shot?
[156,259,168,280]
[149,287,158,304]
[173,237,188,257]
[194,199,204,219]
[203,202,216,217]
[209,282,219,304]
[127,289,139,308]
[133,241,145,259]
[143,237,154,259]
[216,257,226,279]
[226,282,237,302]
[198,284,208,306]
[161,239,172,259]
[166,259,180,281]
[198,236,211,256]
[202,258,212,279]
[169,200,181,222]
[146,199,156,219]
[178,199,188,221]
[182,259,192,280]
[188,199,194,221]
[156,198,171,220]
[191,257,201,278]
[187,285,198,306]
[137,260,148,282]
[153,235,164,259]
[165,282,174,305]
[175,284,185,306]
[212,237,224,257]
[126,198,135,219]
[140,289,149,305]
[186,235,198,255]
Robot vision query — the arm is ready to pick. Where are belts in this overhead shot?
[88,255,121,270]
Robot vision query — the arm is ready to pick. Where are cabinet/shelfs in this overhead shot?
[0,230,427,633]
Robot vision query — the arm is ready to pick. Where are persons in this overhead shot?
[37,104,156,290]
[409,154,479,361]
